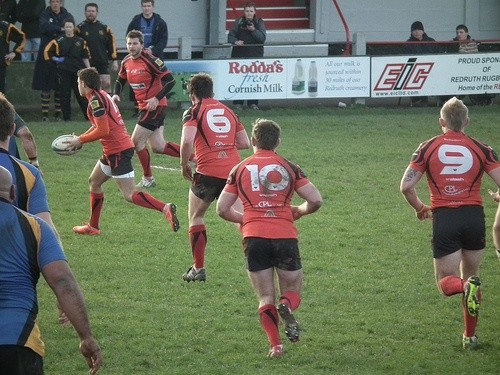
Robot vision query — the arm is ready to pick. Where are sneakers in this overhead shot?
[463,276,482,317]
[73,222,100,235]
[462,333,481,350]
[267,345,283,358]
[181,266,207,284]
[164,203,180,233]
[136,176,156,189]
[276,303,300,342]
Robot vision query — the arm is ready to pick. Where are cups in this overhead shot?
[338,102,346,108]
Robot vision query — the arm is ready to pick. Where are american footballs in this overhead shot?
[50,135,83,157]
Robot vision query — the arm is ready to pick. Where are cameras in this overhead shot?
[241,18,250,27]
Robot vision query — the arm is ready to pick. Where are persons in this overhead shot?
[216,119,322,361]
[180,74,251,283]
[400,97,500,347]
[446,25,486,104]
[0,165,104,375]
[405,21,437,107]
[228,3,266,111]
[0,0,194,325]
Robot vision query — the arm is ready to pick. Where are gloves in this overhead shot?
[29,161,39,168]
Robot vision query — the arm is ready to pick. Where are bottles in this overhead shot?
[307,61,318,97]
[292,58,305,95]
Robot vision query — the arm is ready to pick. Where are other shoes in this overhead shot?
[249,104,260,110]
[235,103,243,111]
[63,117,72,122]
[41,116,50,122]
[55,117,62,121]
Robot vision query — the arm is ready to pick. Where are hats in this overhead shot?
[411,21,425,32]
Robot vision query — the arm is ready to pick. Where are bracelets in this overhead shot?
[415,203,425,214]
